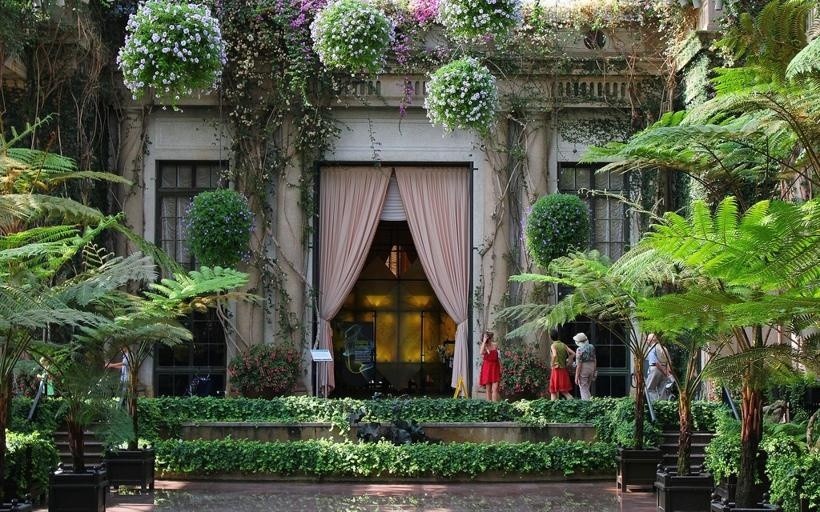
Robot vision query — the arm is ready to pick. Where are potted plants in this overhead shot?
[494,249,680,492]
[0,313,197,512]
[639,191,820,512]
[615,263,737,512]
[64,265,251,493]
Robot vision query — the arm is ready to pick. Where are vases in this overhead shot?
[506,392,539,403]
[243,386,286,400]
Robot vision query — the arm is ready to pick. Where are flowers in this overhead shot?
[228,342,301,391]
[179,189,258,270]
[500,349,551,396]
[114,0,531,137]
[519,193,594,270]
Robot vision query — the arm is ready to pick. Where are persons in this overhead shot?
[103,345,136,385]
[548,329,575,401]
[35,355,56,397]
[646,334,673,403]
[571,332,596,399]
[479,331,502,403]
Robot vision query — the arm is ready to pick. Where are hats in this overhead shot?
[572,332,588,341]
